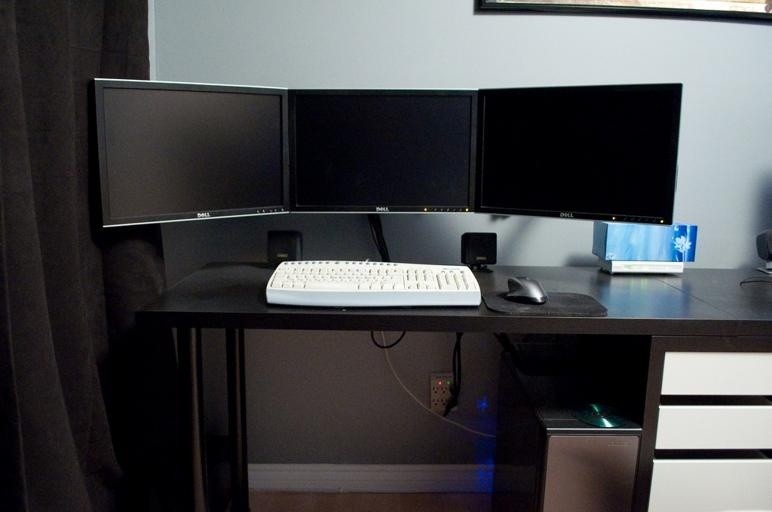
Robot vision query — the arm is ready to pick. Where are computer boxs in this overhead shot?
[490,403,643,512]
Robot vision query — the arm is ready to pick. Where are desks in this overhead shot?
[133,262,771,512]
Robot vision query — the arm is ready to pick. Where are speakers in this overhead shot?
[461,232,497,273]
[268,228,303,269]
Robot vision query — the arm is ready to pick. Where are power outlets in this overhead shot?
[429,370,459,412]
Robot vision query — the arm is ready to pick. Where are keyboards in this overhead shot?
[264,261,482,310]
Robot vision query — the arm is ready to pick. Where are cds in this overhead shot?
[577,403,625,428]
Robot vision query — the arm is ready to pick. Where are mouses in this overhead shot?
[505,277,548,305]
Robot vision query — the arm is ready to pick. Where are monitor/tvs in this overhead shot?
[288,89,478,215]
[474,83,682,225]
[87,77,290,230]
